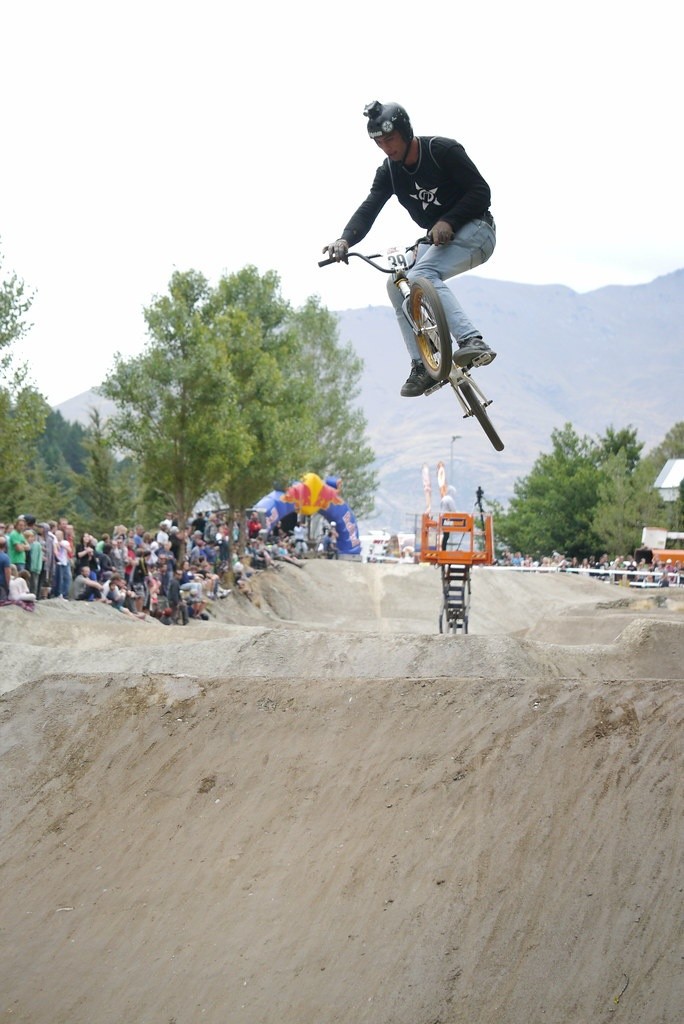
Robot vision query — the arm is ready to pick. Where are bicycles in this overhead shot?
[317,233,504,451]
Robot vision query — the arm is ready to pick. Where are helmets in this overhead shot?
[363,102,407,137]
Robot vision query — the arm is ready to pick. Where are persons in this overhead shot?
[494,551,684,588]
[322,100,498,396]
[381,544,419,564]
[440,485,456,550]
[0,509,339,626]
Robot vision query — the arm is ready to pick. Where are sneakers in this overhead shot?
[452,338,496,367]
[400,362,433,396]
